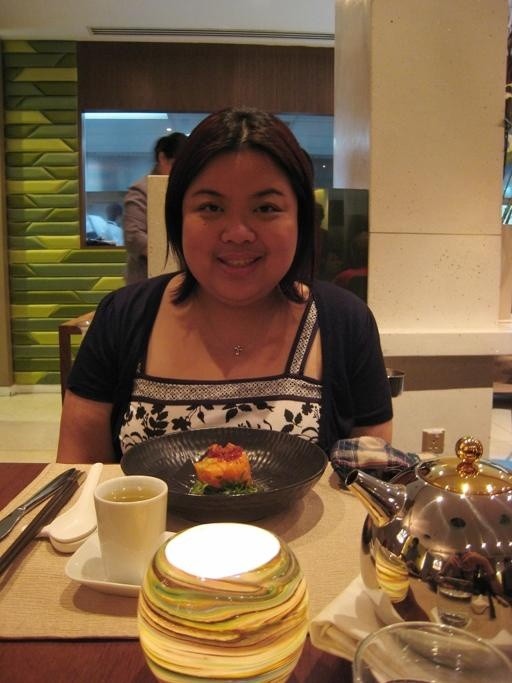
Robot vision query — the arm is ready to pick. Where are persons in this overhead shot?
[55,105,395,466]
[101,199,123,246]
[122,131,188,286]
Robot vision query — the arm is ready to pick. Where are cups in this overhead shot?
[92,475,168,584]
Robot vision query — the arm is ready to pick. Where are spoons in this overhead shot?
[47,462,102,554]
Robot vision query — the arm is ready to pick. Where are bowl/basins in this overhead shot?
[352,620,511,681]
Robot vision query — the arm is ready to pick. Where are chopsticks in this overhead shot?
[0,470,88,574]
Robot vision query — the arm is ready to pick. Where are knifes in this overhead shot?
[0,468,77,541]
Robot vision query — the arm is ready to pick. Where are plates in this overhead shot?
[64,530,171,599]
[121,425,329,524]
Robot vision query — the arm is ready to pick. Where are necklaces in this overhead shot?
[197,293,278,361]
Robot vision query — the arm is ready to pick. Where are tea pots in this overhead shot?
[343,436,512,669]
